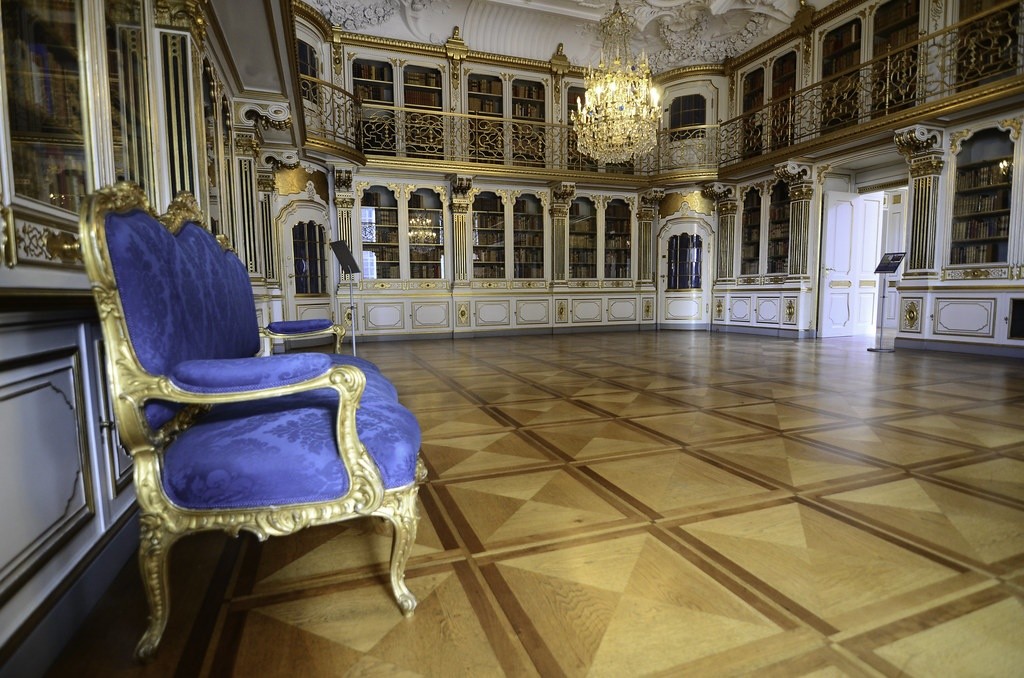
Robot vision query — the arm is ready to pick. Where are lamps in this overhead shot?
[570,0,663,164]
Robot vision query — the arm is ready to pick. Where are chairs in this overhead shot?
[77,180,428,666]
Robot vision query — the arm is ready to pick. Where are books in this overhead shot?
[353,60,395,153]
[958,0,1016,71]
[567,216,597,279]
[471,213,505,278]
[745,56,795,158]
[567,91,599,172]
[469,79,503,165]
[823,22,858,132]
[1,0,85,213]
[362,209,400,279]
[741,191,759,276]
[874,0,913,107]
[406,73,442,145]
[606,215,631,279]
[950,166,1011,264]
[606,163,625,174]
[769,203,789,273]
[512,213,543,278]
[407,211,441,279]
[513,85,545,168]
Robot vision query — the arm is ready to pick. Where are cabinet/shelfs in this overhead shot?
[819,18,863,133]
[353,59,443,130]
[741,200,789,275]
[871,0,920,116]
[467,73,545,159]
[950,155,1015,263]
[568,214,630,280]
[471,210,545,279]
[375,206,442,278]
[743,51,797,158]
